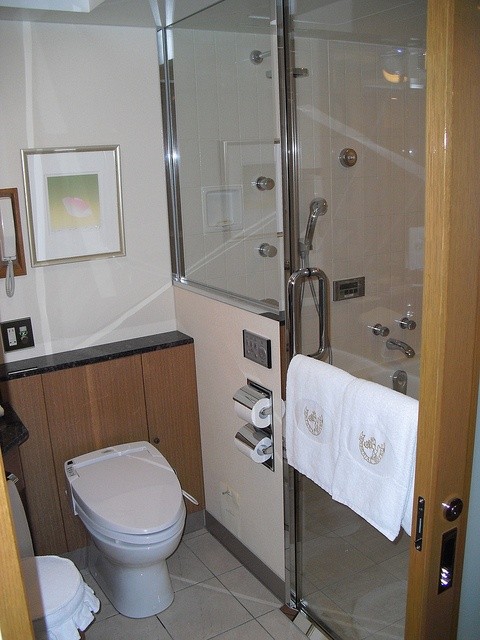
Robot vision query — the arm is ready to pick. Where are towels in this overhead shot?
[285,354,356,497]
[332,378,419,543]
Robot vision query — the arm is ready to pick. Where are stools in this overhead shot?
[21,556,100,640]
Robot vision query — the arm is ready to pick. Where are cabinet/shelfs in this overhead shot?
[0,342,206,556]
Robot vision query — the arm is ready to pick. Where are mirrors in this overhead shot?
[157,0,286,322]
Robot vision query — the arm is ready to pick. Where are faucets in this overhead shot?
[386,337,416,360]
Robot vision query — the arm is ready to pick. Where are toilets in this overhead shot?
[63,440,187,618]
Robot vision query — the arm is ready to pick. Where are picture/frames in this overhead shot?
[219,139,284,245]
[20,144,126,268]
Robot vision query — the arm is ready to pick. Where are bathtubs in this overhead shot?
[348,350,422,401]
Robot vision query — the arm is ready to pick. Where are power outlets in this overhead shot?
[0,317,34,352]
